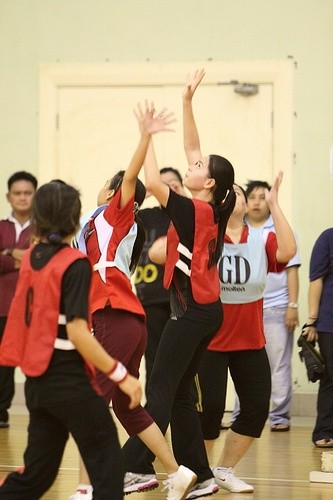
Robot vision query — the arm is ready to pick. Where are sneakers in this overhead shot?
[161,464,198,500]
[212,467,255,494]
[184,476,219,500]
[69,484,94,500]
[122,470,159,495]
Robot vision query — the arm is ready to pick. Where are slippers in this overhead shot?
[315,438,333,448]
[221,420,234,430]
[271,422,291,432]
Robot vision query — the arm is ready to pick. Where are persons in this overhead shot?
[67,107,197,500]
[118,67,237,500]
[135,167,187,409]
[0,171,39,427]
[220,181,301,430]
[0,180,142,500]
[302,227,333,449]
[149,170,297,492]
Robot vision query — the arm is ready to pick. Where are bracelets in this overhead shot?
[7,247,14,255]
[308,316,318,320]
[106,359,129,383]
[287,303,299,309]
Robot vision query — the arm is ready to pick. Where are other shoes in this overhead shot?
[0,409,11,428]
